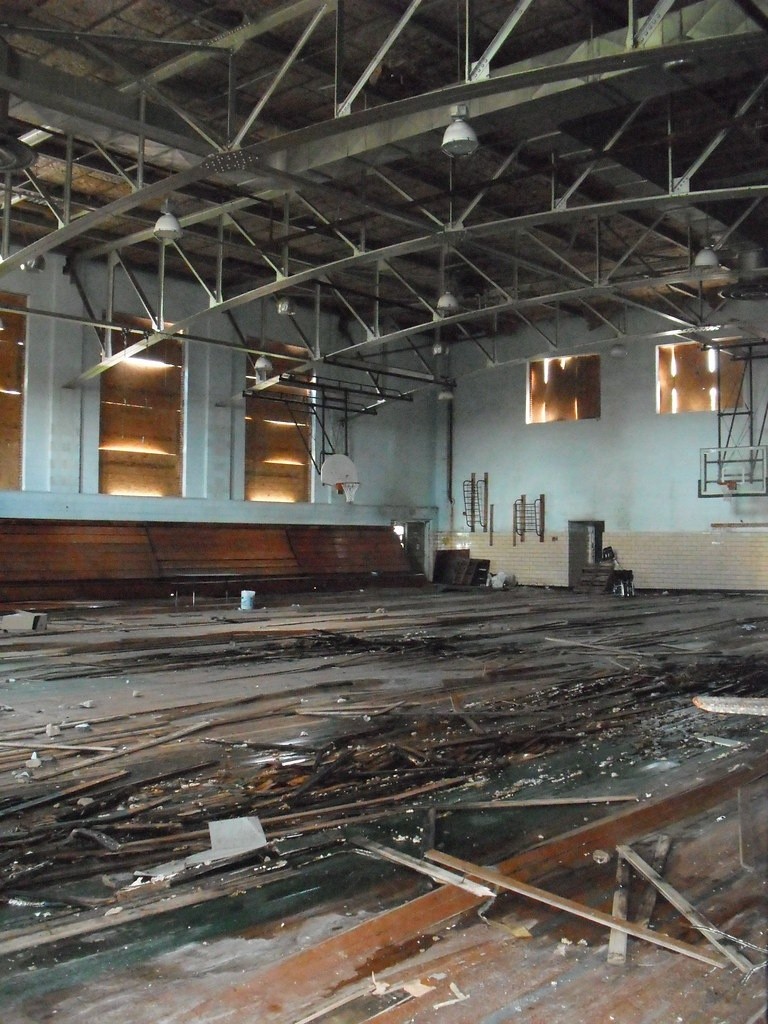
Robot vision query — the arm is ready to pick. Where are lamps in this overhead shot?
[432,342,450,356]
[436,291,459,312]
[663,34,706,76]
[694,246,720,269]
[253,355,273,373]
[152,210,184,241]
[441,104,479,160]
[436,390,455,401]
[276,294,297,316]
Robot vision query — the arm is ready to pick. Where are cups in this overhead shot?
[241,591,256,609]
